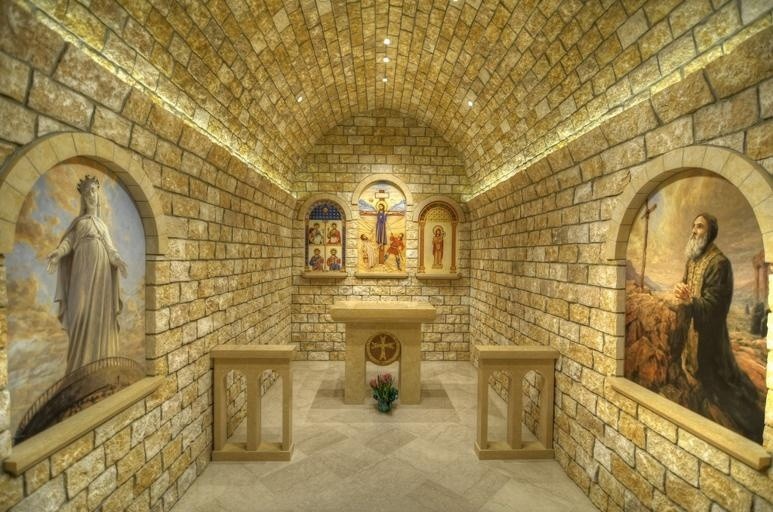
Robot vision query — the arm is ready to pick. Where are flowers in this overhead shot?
[370,373,399,402]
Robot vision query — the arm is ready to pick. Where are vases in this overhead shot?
[377,401,392,412]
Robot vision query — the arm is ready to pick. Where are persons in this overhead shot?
[668,212,765,445]
[359,203,402,246]
[431,228,443,266]
[308,223,341,270]
[383,232,403,271]
[41,173,129,375]
[360,228,378,269]
